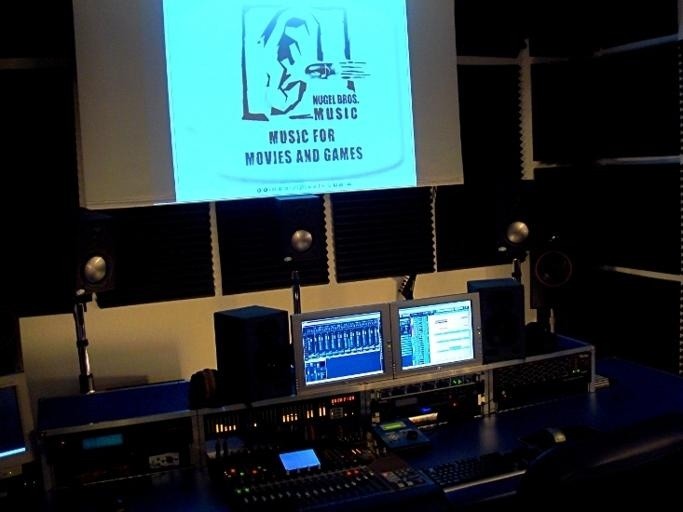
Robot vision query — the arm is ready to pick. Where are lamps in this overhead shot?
[279,221,319,316]
[502,216,534,285]
[69,248,114,394]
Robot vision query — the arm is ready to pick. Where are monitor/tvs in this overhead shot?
[290,302,393,396]
[390,291,484,379]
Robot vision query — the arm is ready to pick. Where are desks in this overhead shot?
[32,334,682,512]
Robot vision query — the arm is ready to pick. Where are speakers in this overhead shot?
[467,277,525,366]
[214,304,294,402]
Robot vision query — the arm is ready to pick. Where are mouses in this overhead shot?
[543,427,566,444]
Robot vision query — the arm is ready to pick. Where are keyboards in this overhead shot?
[421,453,527,494]
